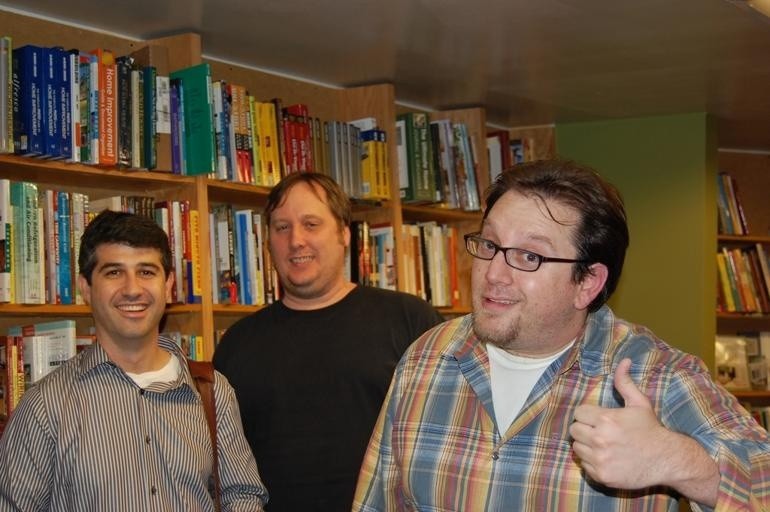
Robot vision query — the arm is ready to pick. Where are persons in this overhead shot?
[0,207,270,512]
[351,160,770,512]
[212,171,447,512]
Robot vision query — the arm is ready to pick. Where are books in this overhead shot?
[1,36,217,175]
[714,172,770,432]
[1,320,96,420]
[343,220,397,290]
[487,130,524,185]
[208,80,390,201]
[0,180,202,305]
[396,111,482,211]
[213,206,280,305]
[402,223,461,308]
[163,328,228,362]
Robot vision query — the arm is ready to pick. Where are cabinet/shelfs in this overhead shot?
[0,33,558,363]
[717,149,770,401]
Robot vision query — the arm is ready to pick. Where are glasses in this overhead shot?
[464,231,594,272]
